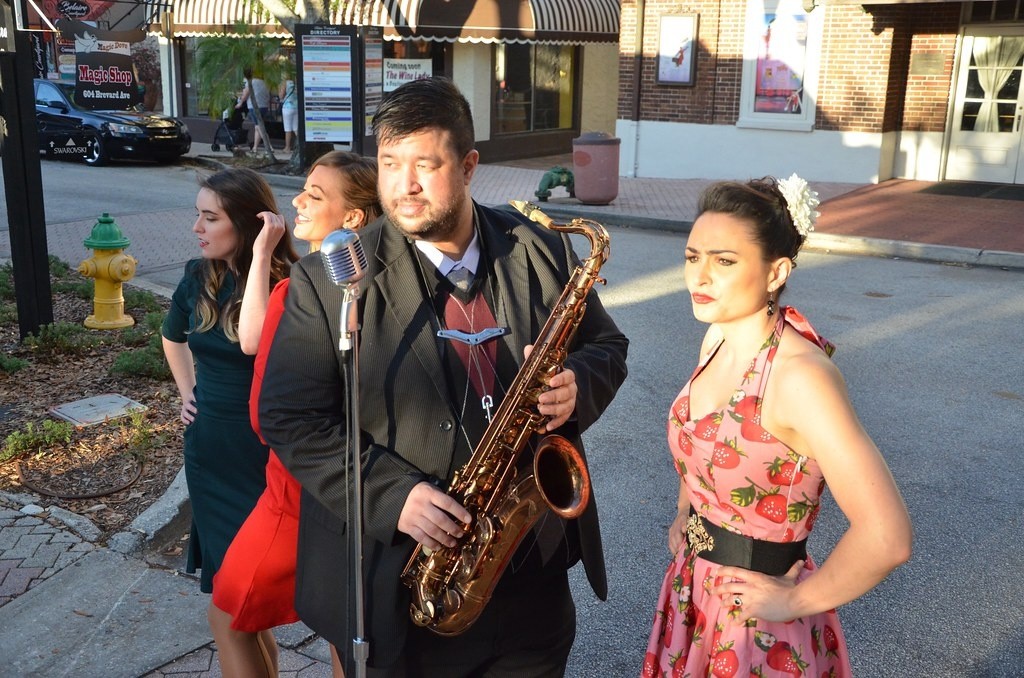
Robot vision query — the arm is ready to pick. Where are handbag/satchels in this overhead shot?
[276,103,283,115]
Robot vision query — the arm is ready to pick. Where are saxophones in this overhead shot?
[402,199,611,634]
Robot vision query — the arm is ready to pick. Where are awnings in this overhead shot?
[143,0,622,47]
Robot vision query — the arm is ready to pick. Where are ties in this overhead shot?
[446,266,474,293]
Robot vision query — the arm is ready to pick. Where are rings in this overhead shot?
[734,594,741,606]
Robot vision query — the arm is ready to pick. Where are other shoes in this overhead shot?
[249,149,258,154]
[264,149,274,155]
[278,149,291,154]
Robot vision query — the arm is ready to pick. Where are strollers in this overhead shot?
[212,93,254,152]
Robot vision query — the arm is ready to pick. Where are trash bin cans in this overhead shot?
[569,130,622,206]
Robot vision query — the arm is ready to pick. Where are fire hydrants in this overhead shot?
[80,211,138,330]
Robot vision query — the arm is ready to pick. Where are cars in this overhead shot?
[0,77,192,166]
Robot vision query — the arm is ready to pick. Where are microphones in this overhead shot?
[320,228,368,362]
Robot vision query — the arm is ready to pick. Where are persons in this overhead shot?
[162,169,303,678]
[279,72,299,154]
[235,67,275,154]
[257,80,630,678]
[207,152,384,678]
[639,177,912,678]
